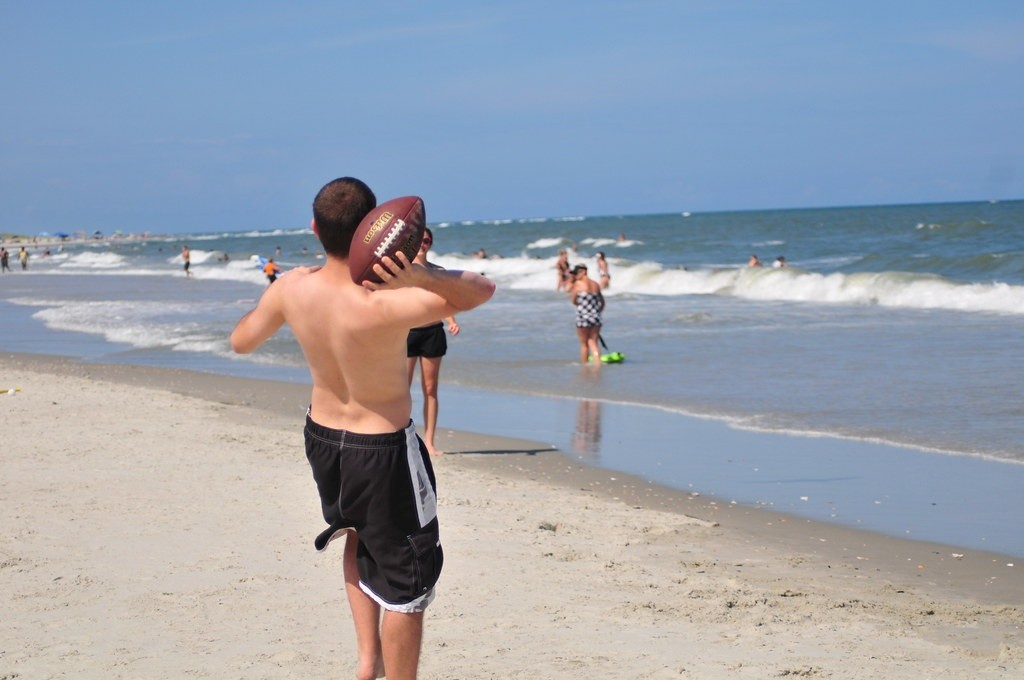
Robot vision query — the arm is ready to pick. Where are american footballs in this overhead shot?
[349,194,426,285]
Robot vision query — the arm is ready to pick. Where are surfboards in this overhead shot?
[250,254,285,276]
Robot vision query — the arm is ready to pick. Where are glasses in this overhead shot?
[573,271,582,275]
[422,238,431,245]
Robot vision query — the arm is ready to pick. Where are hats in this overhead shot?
[569,266,587,274]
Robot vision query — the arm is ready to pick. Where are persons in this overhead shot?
[405,228,460,456]
[264,246,324,284]
[157,245,229,263]
[556,243,621,293]
[231,176,497,680]
[772,256,788,269]
[17,246,29,270]
[619,234,625,242]
[44,244,64,256]
[748,255,763,266]
[0,247,13,273]
[571,263,605,365]
[182,246,192,276]
[469,247,542,260]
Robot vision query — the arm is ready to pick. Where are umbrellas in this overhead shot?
[39,230,151,238]
